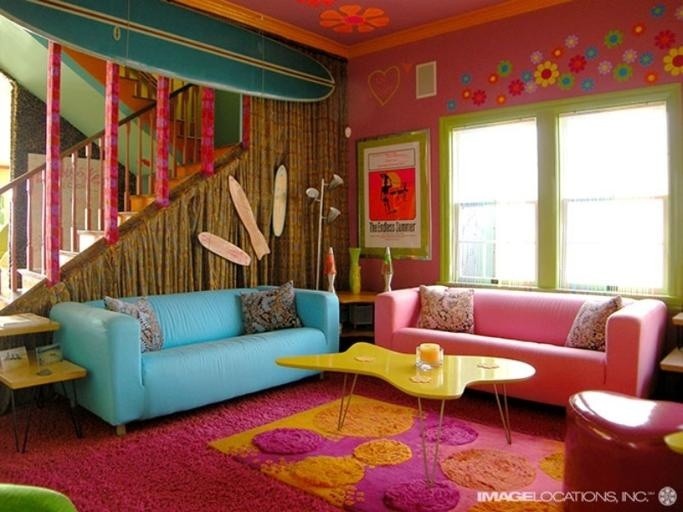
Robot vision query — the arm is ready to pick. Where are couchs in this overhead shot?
[45,283,344,436]
[374,283,670,409]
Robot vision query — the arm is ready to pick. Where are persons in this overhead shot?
[379,174,391,215]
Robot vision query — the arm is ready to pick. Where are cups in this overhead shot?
[416,346,444,372]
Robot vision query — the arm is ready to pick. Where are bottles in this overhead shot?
[348,247,362,295]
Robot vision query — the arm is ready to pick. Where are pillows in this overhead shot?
[563,294,622,351]
[101,295,162,354]
[414,284,475,335]
[238,281,305,335]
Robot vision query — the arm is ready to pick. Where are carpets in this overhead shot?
[206,392,563,511]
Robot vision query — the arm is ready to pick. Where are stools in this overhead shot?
[562,389,682,512]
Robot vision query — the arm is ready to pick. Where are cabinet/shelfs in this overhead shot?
[0,310,87,452]
[337,290,377,336]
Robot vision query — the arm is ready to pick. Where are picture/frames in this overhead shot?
[355,128,432,262]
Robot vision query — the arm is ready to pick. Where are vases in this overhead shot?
[348,247,361,294]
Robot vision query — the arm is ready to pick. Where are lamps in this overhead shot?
[304,173,344,290]
